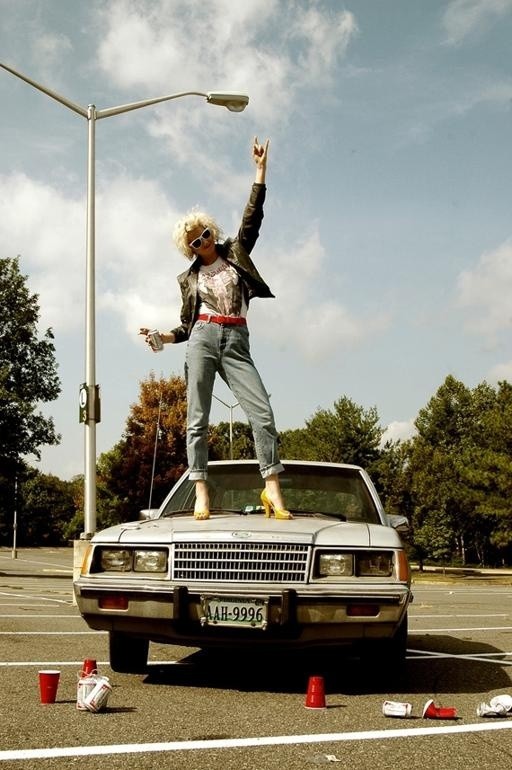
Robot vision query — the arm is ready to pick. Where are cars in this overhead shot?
[71,456,415,690]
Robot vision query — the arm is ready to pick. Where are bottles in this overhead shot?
[77,658,113,711]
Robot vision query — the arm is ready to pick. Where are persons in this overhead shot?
[137,135,299,520]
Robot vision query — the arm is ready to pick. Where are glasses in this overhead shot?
[189,228,211,250]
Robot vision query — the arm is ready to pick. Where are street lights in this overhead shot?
[211,392,273,509]
[3,61,249,611]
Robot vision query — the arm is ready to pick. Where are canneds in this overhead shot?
[477,694,512,718]
[382,700,415,717]
[147,329,164,354]
[75,675,112,713]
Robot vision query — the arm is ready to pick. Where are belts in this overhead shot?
[197,314,246,325]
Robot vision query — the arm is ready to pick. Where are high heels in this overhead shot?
[261,488,293,519]
[193,498,211,519]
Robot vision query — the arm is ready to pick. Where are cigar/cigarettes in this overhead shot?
[138,329,147,335]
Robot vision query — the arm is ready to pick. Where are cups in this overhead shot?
[38,670,61,704]
[382,696,512,719]
[305,676,326,710]
